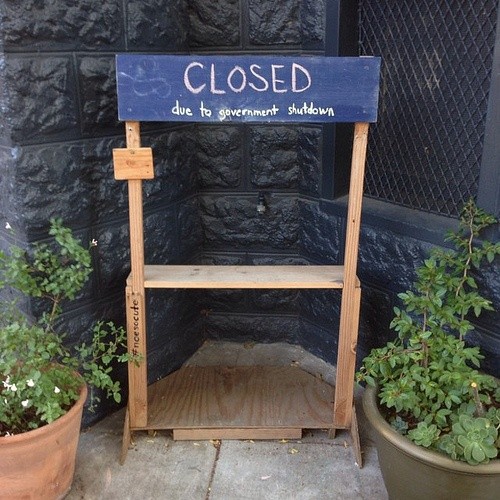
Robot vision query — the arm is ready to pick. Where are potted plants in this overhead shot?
[352,200,500,500]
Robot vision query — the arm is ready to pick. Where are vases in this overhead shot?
[1,361,89,499]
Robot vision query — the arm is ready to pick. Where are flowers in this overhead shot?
[1,217,144,434]
